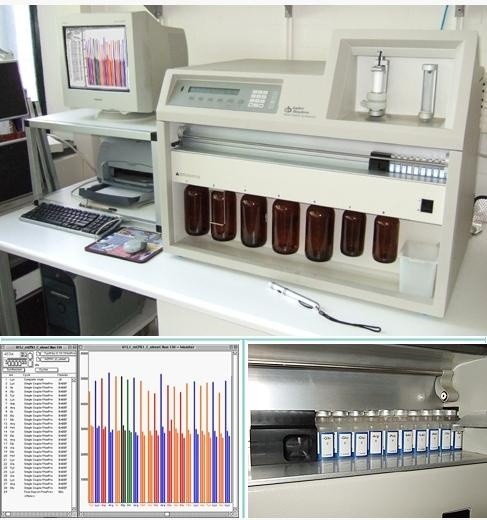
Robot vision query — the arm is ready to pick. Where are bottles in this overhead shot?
[318,410,464,458]
[184,186,400,263]
[388,155,448,180]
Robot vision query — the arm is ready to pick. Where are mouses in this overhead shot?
[123,239,147,253]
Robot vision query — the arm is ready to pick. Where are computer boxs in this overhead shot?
[38,262,145,336]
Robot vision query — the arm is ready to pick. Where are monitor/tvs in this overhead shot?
[53,11,188,122]
[0,58,29,122]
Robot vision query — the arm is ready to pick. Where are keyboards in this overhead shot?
[19,202,121,239]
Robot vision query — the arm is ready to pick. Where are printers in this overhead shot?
[79,135,154,208]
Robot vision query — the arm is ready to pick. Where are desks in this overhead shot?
[0,177,487,338]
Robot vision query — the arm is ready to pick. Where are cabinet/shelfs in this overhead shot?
[23,107,159,235]
